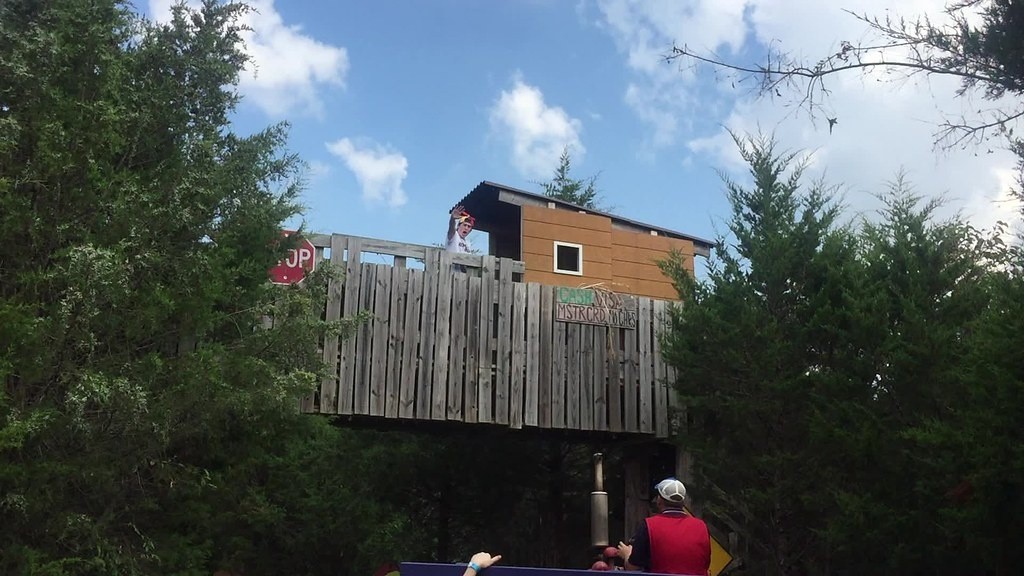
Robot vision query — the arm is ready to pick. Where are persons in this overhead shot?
[618,478,712,576]
[462,552,502,576]
[445,204,475,255]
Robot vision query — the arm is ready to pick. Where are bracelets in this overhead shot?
[467,562,481,573]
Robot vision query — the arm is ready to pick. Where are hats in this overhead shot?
[457,216,475,228]
[592,560,609,570]
[655,477,687,503]
[603,547,618,558]
